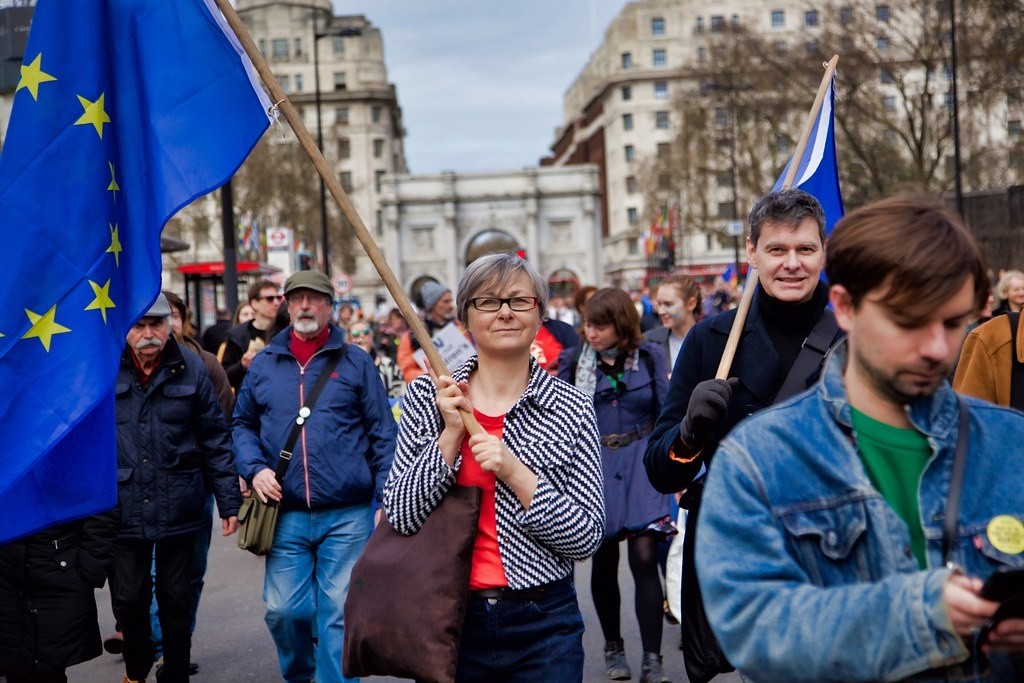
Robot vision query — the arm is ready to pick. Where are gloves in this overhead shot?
[678,377,739,447]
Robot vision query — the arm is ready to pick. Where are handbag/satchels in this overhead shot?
[341,482,483,683]
[235,488,280,556]
[410,321,477,377]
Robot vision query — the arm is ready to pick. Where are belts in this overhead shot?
[600,423,654,449]
[469,586,526,598]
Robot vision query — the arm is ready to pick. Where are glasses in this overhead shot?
[258,295,283,302]
[464,296,540,312]
[351,329,370,337]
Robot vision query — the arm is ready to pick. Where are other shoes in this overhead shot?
[188,663,199,675]
[103,631,126,654]
[604,649,631,681]
[639,652,672,683]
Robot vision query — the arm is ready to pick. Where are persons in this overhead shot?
[0,189,1024,683]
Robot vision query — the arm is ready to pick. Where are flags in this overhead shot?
[745,54,845,310]
[0,0,280,537]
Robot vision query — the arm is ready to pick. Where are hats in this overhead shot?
[143,292,171,317]
[283,269,336,299]
[420,281,451,311]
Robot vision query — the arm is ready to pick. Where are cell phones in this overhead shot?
[977,564,1024,625]
